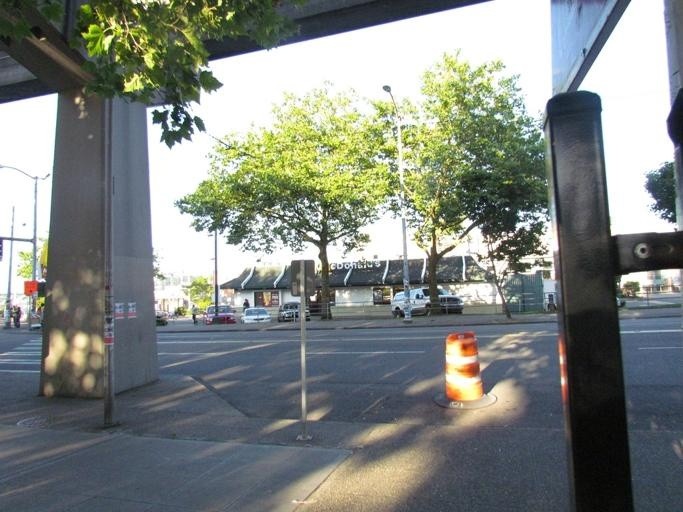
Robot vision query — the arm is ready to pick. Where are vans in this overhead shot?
[391,288,463,316]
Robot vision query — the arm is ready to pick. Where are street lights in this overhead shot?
[382,85,409,288]
[0,165,50,312]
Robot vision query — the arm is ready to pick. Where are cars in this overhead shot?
[203,304,236,323]
[278,301,310,321]
[240,307,272,322]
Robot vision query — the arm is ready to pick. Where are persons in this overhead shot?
[0,302,23,328]
[191,305,197,324]
[242,299,249,312]
